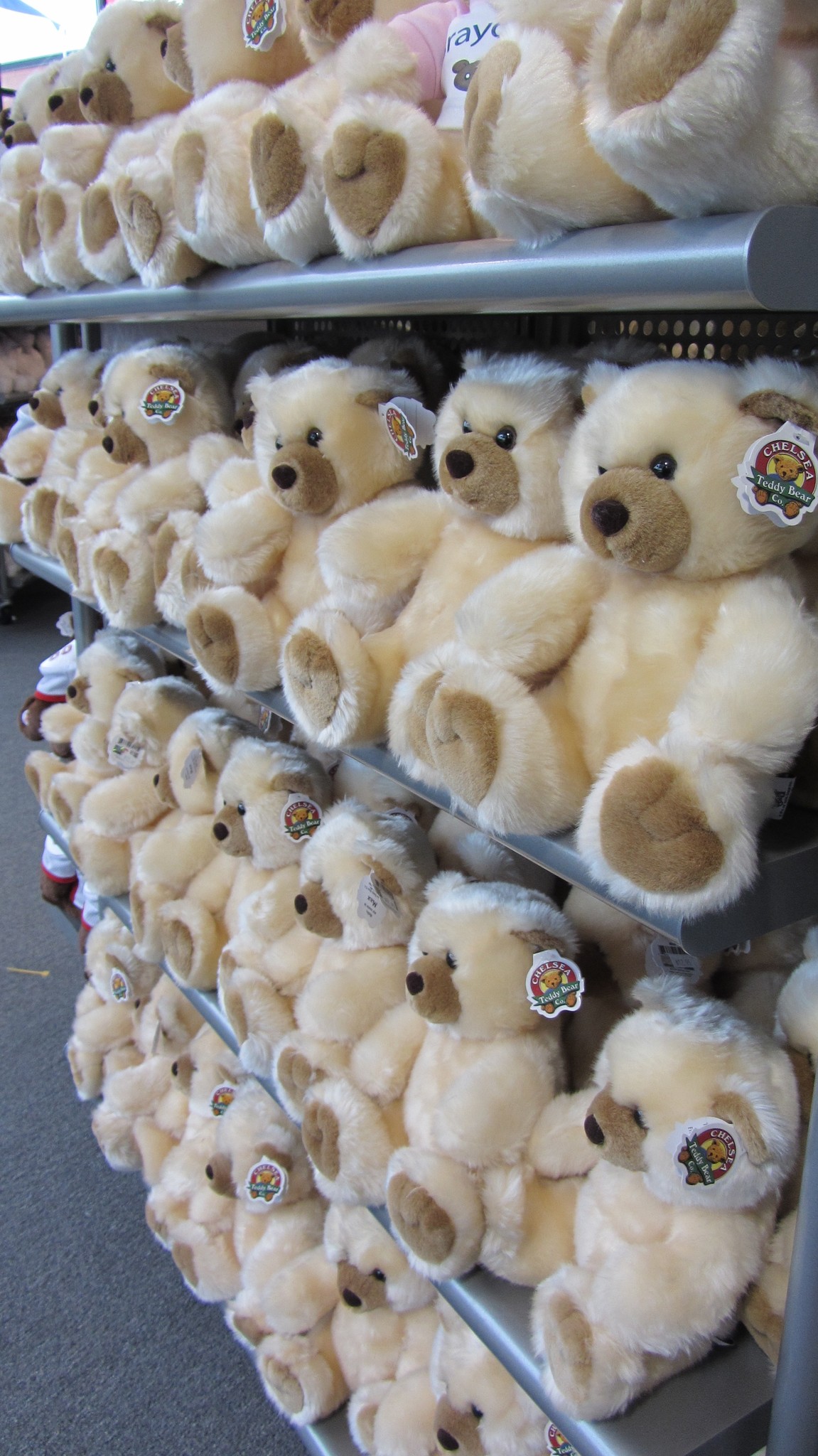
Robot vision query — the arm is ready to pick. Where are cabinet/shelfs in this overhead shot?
[0,198,818,1456]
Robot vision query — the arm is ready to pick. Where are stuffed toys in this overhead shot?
[427,356,816,924]
[26,634,818,1456]
[281,349,587,786]
[177,358,439,695]
[1,327,323,633]
[0,1,816,300]
